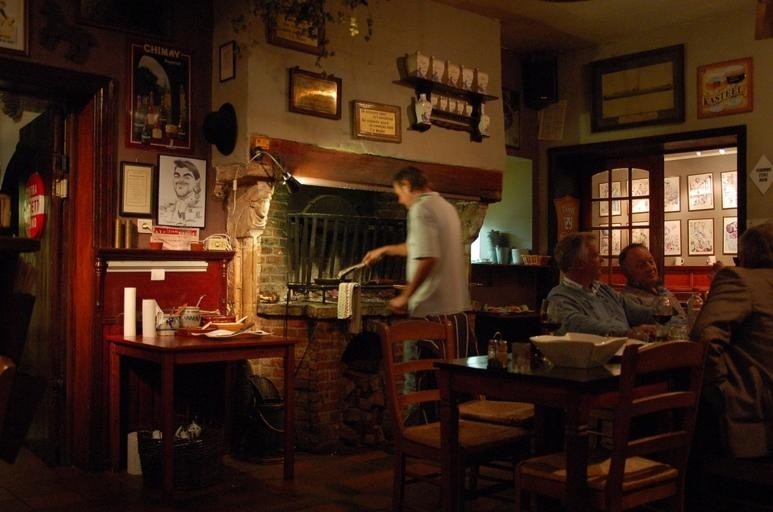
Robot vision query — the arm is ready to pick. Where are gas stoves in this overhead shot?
[288,283,396,293]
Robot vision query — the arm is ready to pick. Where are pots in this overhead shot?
[314,262,366,285]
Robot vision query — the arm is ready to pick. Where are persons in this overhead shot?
[362,167,471,439]
[160,159,203,225]
[688,220,773,465]
[619,242,689,327]
[547,232,666,339]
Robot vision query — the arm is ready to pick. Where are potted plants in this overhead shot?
[489,229,510,264]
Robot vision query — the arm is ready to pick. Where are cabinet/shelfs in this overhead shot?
[406,75,500,141]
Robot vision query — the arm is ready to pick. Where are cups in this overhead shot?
[487,340,507,367]
[512,343,531,370]
[182,307,200,327]
[160,314,177,334]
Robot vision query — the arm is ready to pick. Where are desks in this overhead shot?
[105,328,298,491]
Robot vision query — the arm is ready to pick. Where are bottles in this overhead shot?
[132,84,187,146]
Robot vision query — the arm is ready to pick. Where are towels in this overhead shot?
[338,279,365,334]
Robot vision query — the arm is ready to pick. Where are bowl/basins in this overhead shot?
[531,333,627,369]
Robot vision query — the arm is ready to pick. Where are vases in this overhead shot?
[512,246,529,264]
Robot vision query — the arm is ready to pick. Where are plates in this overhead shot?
[204,329,247,339]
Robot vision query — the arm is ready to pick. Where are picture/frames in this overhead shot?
[219,39,237,80]
[120,153,207,227]
[267,4,327,56]
[351,97,405,143]
[0,1,34,58]
[288,67,344,121]
[696,55,753,119]
